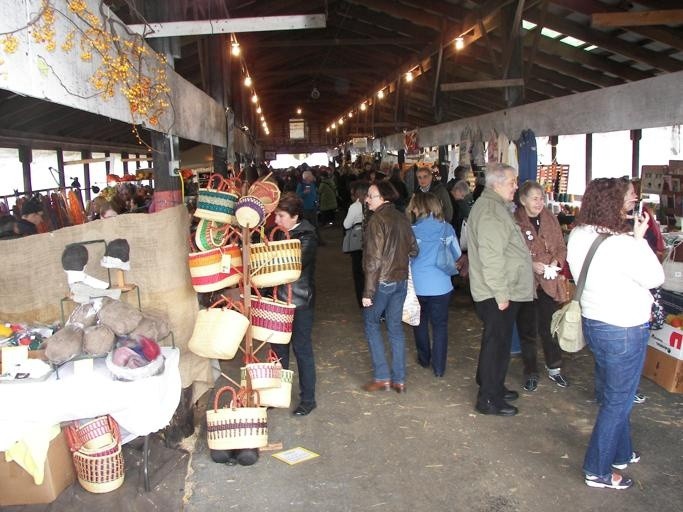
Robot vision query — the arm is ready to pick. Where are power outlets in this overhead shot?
[168,161,179,176]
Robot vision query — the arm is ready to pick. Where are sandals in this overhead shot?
[586,472,634,489]
[612,450,641,470]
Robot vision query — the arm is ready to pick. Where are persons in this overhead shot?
[568,178,666,491]
[99,202,119,218]
[121,184,150,213]
[408,191,462,378]
[318,171,338,229]
[390,167,410,212]
[416,167,453,222]
[361,182,421,393]
[466,163,536,417]
[513,179,573,392]
[619,176,657,257]
[337,156,373,180]
[371,171,400,205]
[446,165,477,193]
[296,170,317,222]
[91,196,108,220]
[188,175,199,195]
[310,161,339,179]
[271,163,313,193]
[343,180,364,307]
[0,215,26,240]
[262,194,318,417]
[338,168,359,219]
[450,180,470,243]
[16,199,45,235]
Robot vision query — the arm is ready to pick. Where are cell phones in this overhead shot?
[633,198,643,217]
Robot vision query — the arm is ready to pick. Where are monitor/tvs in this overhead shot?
[264,150,276,160]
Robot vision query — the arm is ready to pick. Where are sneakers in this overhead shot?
[524,376,542,393]
[549,372,571,387]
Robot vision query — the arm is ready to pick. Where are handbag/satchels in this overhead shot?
[195,175,237,224]
[206,386,267,450]
[235,197,265,228]
[402,247,421,326]
[194,216,231,250]
[65,416,125,493]
[189,230,243,293]
[249,181,281,214]
[188,298,250,360]
[240,365,293,409]
[343,200,366,251]
[249,227,302,288]
[550,233,615,352]
[238,277,296,345]
[246,351,282,389]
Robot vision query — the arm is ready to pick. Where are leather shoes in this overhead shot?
[390,382,405,392]
[363,376,391,392]
[500,388,519,398]
[478,400,517,417]
[294,399,316,414]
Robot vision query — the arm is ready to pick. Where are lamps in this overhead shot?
[49,167,81,188]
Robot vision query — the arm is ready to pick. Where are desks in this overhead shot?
[0,349,180,495]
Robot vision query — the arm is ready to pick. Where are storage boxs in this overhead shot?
[0,431,77,506]
[647,322,682,360]
[642,347,683,393]
